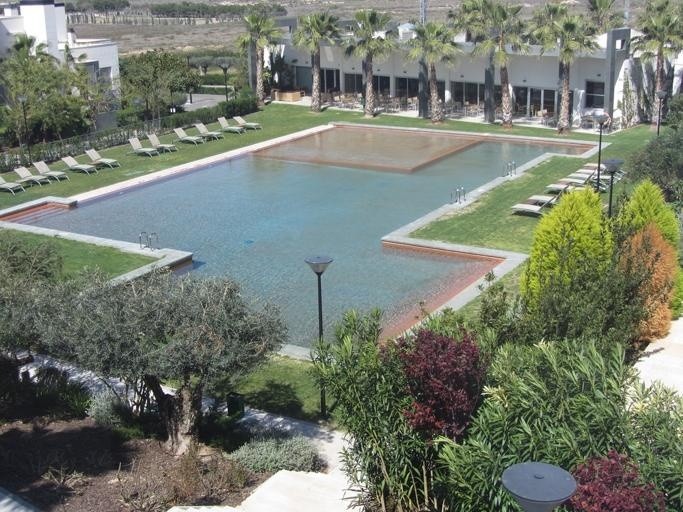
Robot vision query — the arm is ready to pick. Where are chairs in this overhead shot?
[0,115,262,195]
[509,161,626,216]
[331,87,548,122]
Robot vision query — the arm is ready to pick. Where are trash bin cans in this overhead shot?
[226,391,245,418]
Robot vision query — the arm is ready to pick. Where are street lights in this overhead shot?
[215,59,232,101]
[184,45,196,104]
[654,90,668,139]
[18,93,33,166]
[301,252,336,415]
[603,158,626,217]
[589,113,610,193]
[500,461,578,511]
